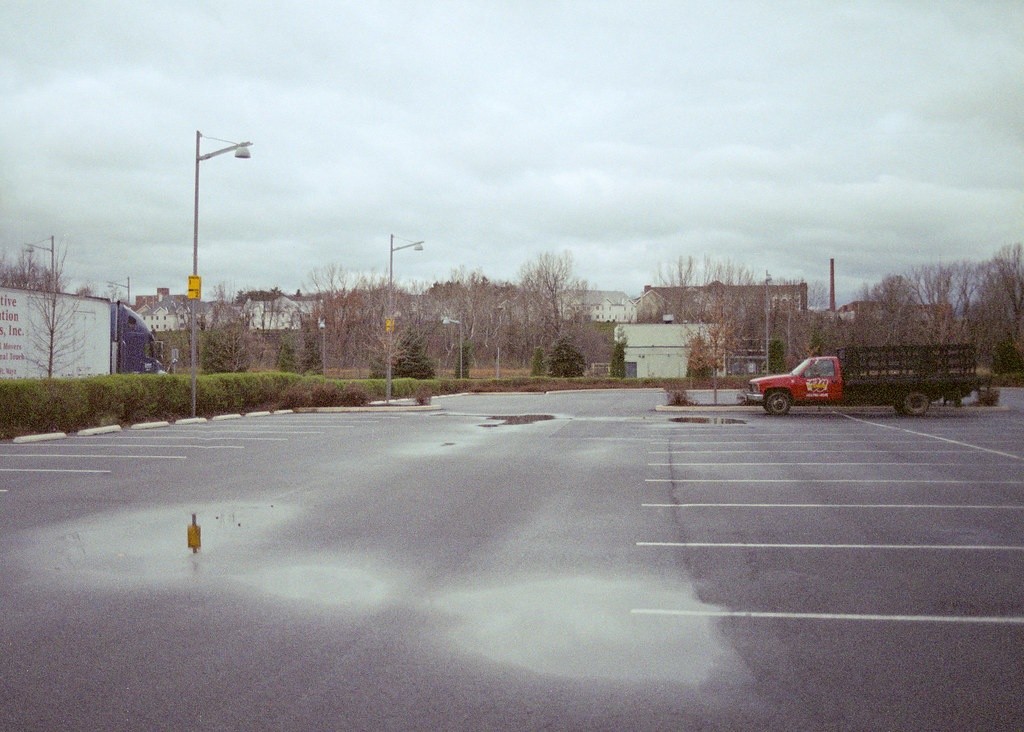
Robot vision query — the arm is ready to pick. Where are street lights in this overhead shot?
[765,269,772,376]
[386,233,425,401]
[188,130,253,419]
[26,235,54,291]
[107,276,130,306]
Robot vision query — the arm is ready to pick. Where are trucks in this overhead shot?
[746,346,979,418]
[0,285,159,375]
